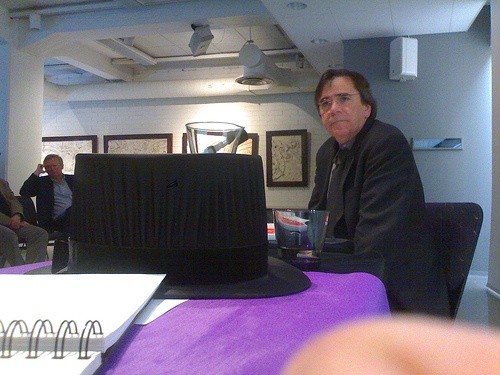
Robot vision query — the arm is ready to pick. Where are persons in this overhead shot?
[19,154,75,237]
[281,312,500,375]
[306,68,443,317]
[0,178,48,268]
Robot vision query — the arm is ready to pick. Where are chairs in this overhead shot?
[426,202,484,320]
[16,196,71,260]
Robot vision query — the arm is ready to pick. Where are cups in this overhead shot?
[271,207,330,264]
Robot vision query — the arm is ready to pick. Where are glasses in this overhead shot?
[318,93,361,111]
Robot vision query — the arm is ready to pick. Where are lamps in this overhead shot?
[97,38,158,65]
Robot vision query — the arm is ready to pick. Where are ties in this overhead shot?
[323,147,349,239]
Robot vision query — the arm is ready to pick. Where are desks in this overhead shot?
[0,261,390,375]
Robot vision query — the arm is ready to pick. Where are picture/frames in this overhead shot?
[42,135,97,176]
[182,133,258,155]
[266,129,308,187]
[104,133,173,154]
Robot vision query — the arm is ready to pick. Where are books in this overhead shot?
[0,274,167,375]
